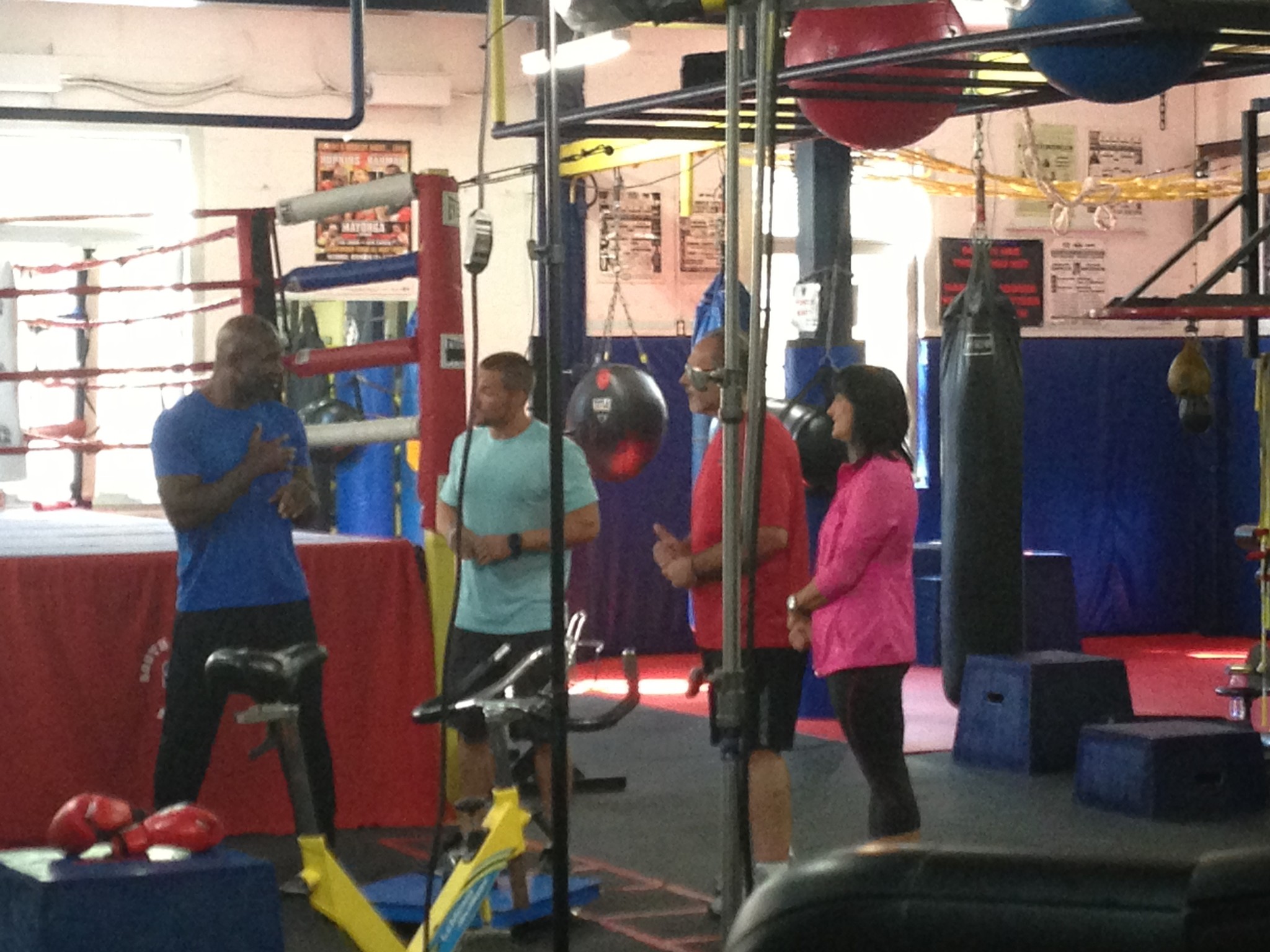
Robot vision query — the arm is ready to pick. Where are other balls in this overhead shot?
[784,0,971,152]
[1006,0,1234,106]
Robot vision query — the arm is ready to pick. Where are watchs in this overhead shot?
[508,532,522,556]
[787,593,810,617]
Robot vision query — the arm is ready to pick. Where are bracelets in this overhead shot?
[691,553,707,579]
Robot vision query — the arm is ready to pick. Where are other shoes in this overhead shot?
[714,871,726,893]
[707,865,769,920]
[443,846,476,880]
[540,847,573,873]
[279,857,348,893]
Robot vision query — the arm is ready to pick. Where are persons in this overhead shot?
[654,328,811,918]
[153,315,338,850]
[435,350,601,877]
[787,365,922,844]
[317,160,411,247]
